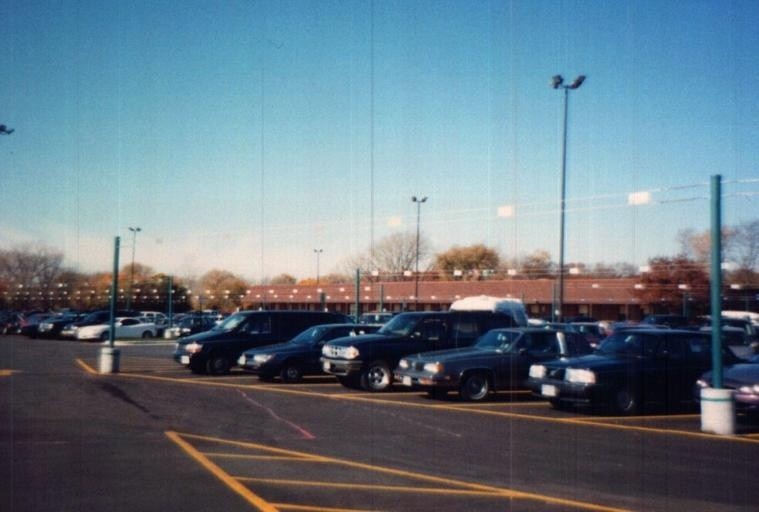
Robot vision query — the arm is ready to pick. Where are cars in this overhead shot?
[235,322,385,385]
[346,294,759,363]
[0,305,230,346]
[391,325,595,403]
[689,351,759,428]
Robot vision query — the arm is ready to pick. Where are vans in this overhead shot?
[173,309,356,377]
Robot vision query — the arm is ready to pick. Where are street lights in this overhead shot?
[124,221,145,313]
[407,195,429,307]
[549,74,591,322]
[311,246,325,284]
[0,123,15,139]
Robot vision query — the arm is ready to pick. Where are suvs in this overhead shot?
[318,309,521,393]
[525,324,738,418]
